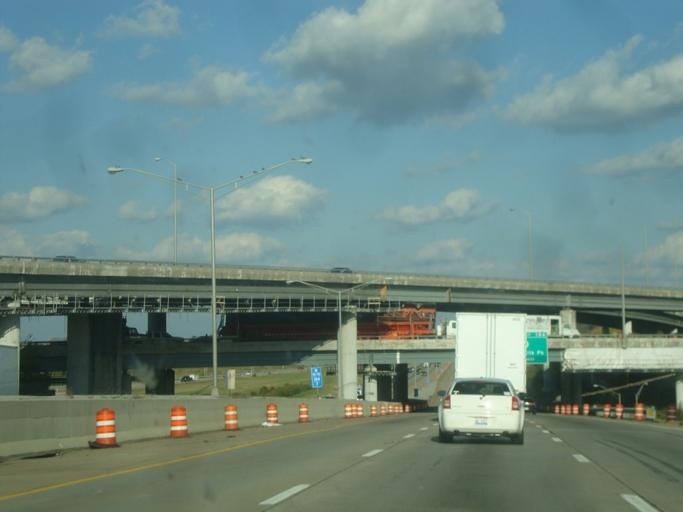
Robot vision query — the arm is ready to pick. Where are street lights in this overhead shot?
[591,383,622,405]
[153,153,178,265]
[101,156,315,393]
[506,206,536,279]
[633,382,650,405]
[607,196,630,349]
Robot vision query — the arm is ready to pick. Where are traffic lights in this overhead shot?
[309,366,323,388]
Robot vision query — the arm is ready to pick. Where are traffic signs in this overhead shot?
[524,332,547,366]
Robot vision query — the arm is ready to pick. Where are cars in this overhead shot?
[327,264,354,274]
[517,391,537,415]
[432,375,527,447]
[407,361,440,377]
[319,384,364,401]
[180,373,199,383]
[53,255,80,263]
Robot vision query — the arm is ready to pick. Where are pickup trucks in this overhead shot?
[125,327,148,339]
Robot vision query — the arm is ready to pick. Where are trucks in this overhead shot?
[453,308,528,396]
